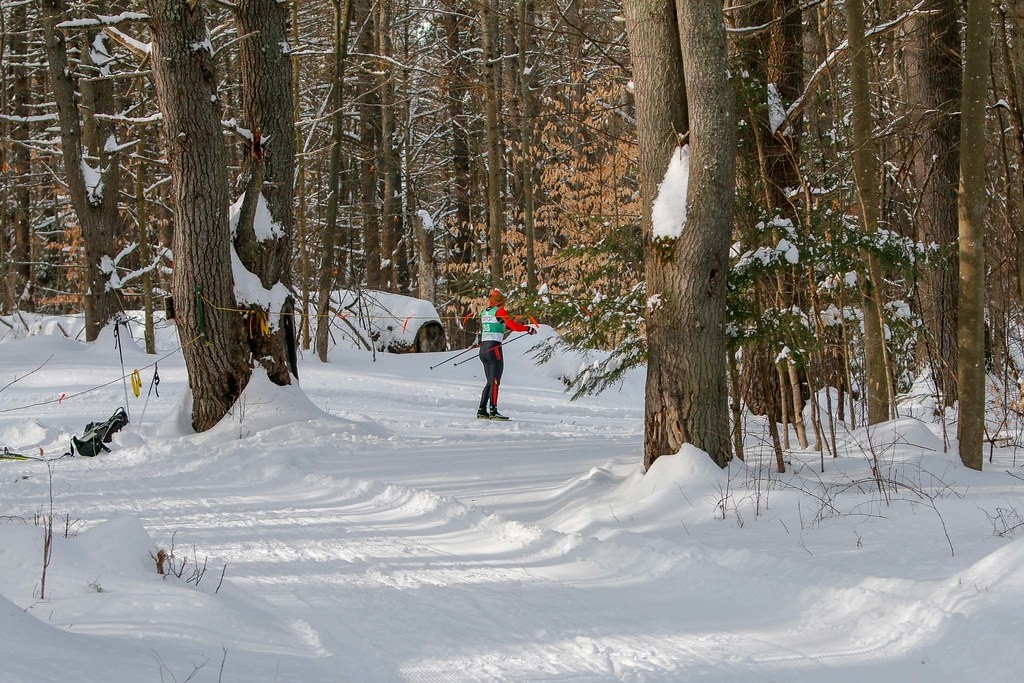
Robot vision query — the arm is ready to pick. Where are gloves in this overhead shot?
[528,326,537,335]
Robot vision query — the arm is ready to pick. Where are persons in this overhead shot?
[476,290,538,419]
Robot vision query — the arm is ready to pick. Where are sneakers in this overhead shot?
[476,407,489,419]
[489,409,510,421]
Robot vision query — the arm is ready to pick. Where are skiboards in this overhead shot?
[476,415,577,424]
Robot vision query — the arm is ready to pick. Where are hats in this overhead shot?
[489,288,505,308]
